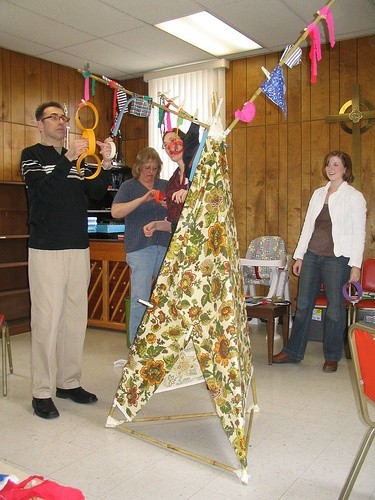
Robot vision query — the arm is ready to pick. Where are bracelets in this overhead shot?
[100,160,112,171]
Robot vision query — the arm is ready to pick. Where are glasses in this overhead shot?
[162,137,180,149]
[142,165,159,173]
[41,114,70,122]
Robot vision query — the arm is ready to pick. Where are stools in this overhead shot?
[0,313,14,396]
[246,303,290,365]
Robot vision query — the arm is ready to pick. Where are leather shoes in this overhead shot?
[32,397,59,418]
[323,360,337,372]
[272,351,300,364]
[56,386,98,403]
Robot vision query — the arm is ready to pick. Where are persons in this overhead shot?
[111,128,190,349]
[271,150,367,373]
[21,102,114,419]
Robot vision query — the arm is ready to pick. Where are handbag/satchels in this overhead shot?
[0,475,84,500]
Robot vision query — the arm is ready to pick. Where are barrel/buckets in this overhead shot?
[124,296,132,349]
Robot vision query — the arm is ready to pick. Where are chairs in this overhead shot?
[315,258,375,500]
[240,235,293,339]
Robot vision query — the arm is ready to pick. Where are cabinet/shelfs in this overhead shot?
[0,181,32,338]
[87,242,131,331]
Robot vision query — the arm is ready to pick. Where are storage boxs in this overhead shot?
[309,309,327,342]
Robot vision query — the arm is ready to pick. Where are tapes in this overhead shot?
[103,141,116,159]
